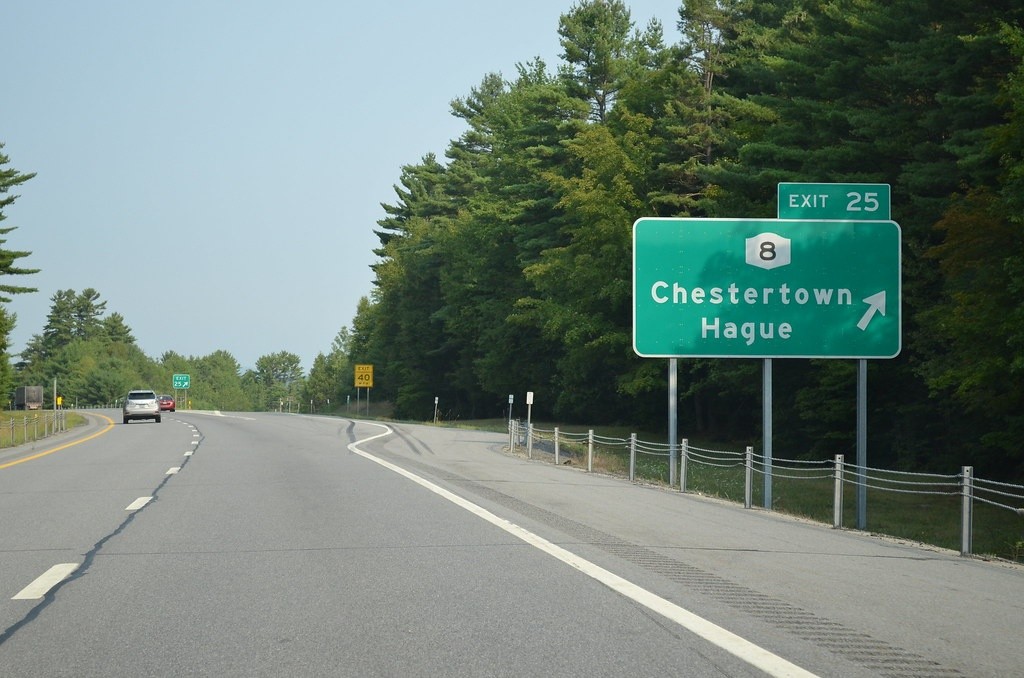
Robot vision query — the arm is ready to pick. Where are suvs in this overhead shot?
[123,389,162,424]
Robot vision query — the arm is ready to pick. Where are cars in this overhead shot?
[157,395,175,412]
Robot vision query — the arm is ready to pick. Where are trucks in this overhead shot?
[14,385,44,410]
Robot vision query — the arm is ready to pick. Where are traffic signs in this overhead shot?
[633,219,902,358]
[173,374,190,389]
